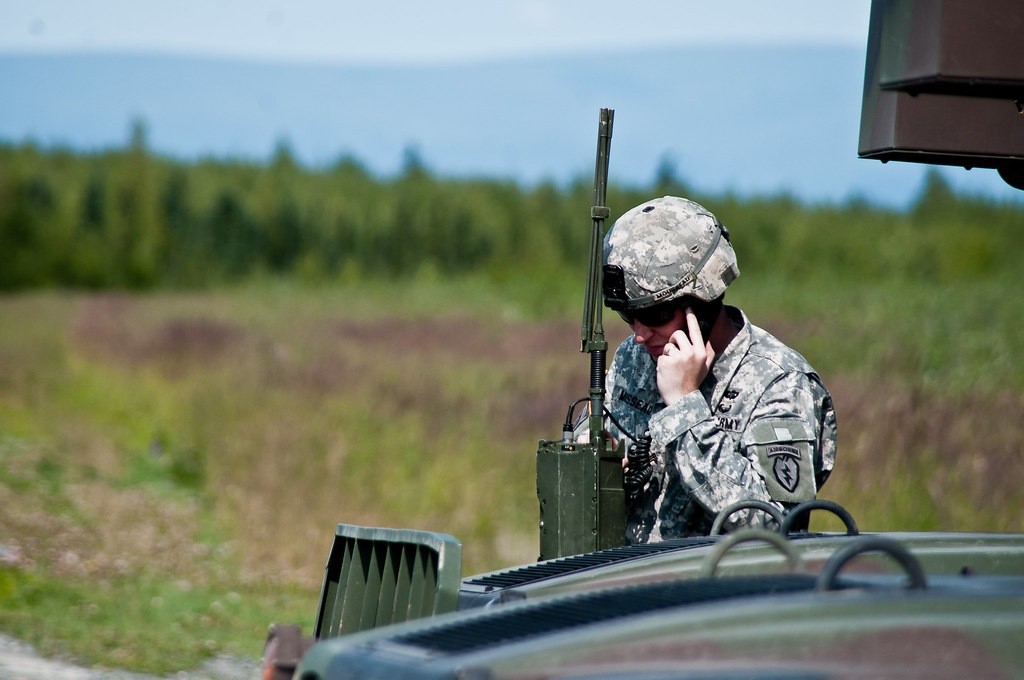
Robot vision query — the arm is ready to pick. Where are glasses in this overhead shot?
[617,297,693,326]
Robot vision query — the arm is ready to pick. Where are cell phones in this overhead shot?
[676,296,723,352]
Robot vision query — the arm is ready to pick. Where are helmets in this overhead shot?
[602,195,741,312]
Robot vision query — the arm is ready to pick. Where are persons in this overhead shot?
[573,196,839,544]
